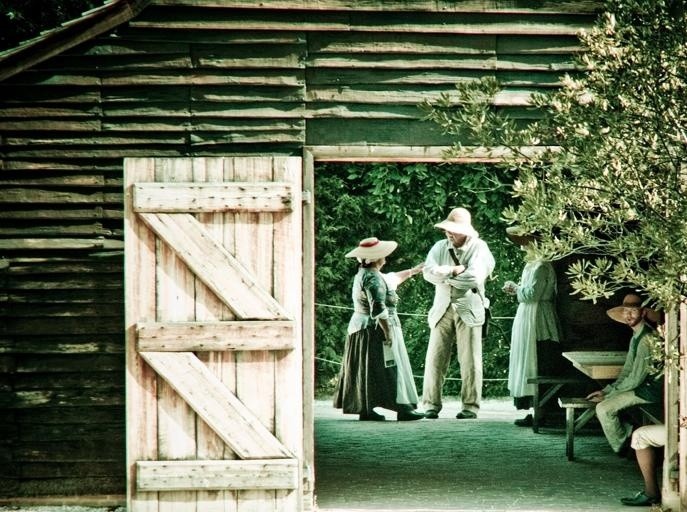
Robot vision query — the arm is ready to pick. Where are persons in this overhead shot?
[501,227,563,426]
[415,208,495,419]
[585,293,662,453]
[331,237,425,422]
[620,424,667,504]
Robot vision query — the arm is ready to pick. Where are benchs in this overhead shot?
[526,375,664,460]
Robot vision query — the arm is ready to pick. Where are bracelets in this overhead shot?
[408,268,412,277]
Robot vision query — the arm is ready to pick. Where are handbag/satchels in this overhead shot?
[382,342,397,368]
[482,307,490,337]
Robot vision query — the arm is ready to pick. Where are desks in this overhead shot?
[560,351,641,425]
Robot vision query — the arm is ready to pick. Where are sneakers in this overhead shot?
[359,412,386,421]
[514,414,535,429]
[397,410,439,420]
[456,410,476,419]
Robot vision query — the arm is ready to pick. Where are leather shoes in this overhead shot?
[620,492,662,507]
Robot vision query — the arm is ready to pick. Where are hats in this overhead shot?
[434,207,479,237]
[344,237,397,259]
[505,224,523,236]
[606,294,661,325]
